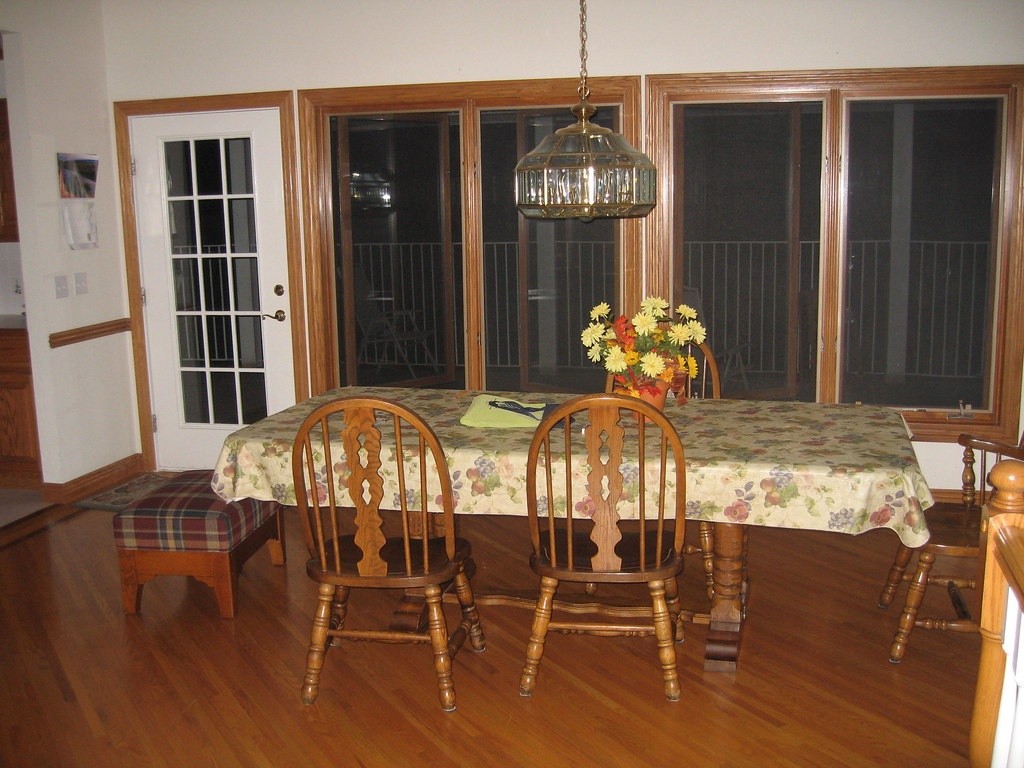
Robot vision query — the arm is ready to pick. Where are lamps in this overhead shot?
[513,0,657,221]
[348,129,397,217]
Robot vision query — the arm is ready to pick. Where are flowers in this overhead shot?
[582,295,707,398]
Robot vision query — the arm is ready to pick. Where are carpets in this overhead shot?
[73,470,172,512]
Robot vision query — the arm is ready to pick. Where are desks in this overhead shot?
[211,385,936,673]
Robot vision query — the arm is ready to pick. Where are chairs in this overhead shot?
[605,321,721,603]
[292,396,487,713]
[518,393,687,701]
[878,430,1024,665]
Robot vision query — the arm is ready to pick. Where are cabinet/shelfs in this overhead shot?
[0,328,40,491]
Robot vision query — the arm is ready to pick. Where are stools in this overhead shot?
[111,469,288,620]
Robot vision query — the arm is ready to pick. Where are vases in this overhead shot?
[630,379,671,423]
